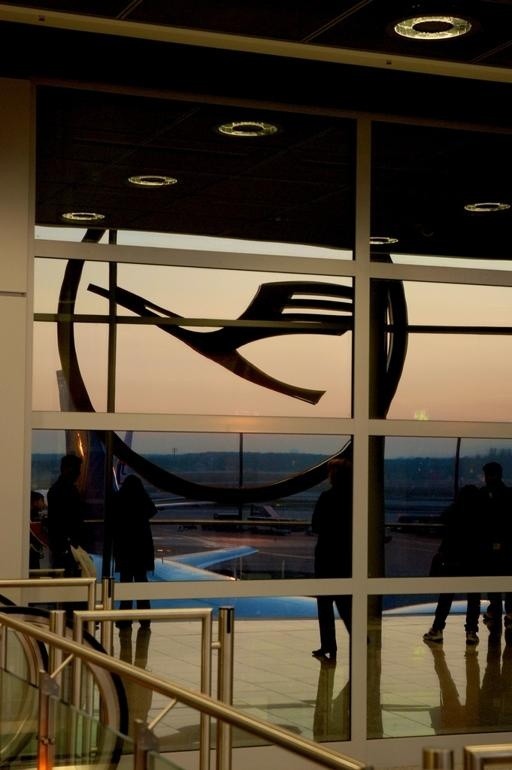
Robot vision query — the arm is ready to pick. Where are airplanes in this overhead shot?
[33,369,291,536]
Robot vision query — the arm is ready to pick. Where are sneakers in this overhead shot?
[504,615,511,621]
[483,612,502,619]
[423,628,443,640]
[466,632,478,644]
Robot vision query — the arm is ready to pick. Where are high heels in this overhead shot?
[313,647,337,655]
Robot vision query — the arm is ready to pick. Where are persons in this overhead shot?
[312,457,351,657]
[46,454,99,629]
[31,492,45,578]
[423,484,489,644]
[111,473,156,627]
[479,460,512,621]
[418,639,511,734]
